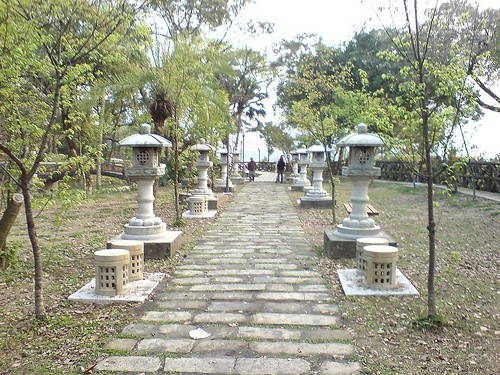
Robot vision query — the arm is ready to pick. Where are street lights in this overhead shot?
[258,148,261,162]
[241,132,245,180]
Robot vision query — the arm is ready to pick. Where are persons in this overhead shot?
[276,155,285,183]
[248,158,256,182]
